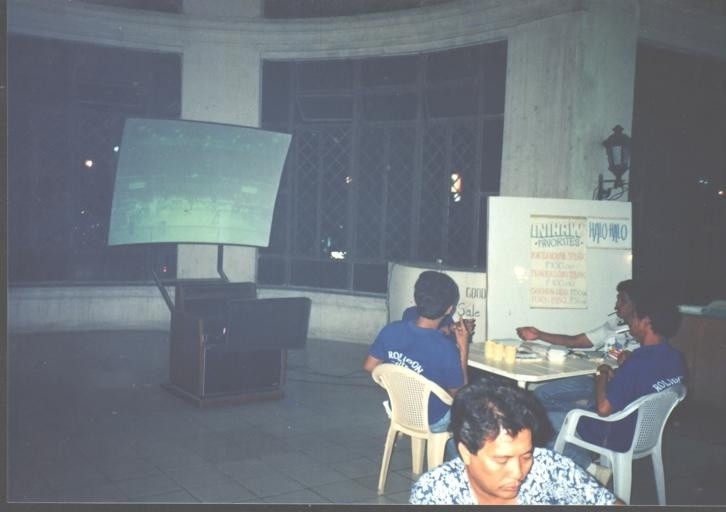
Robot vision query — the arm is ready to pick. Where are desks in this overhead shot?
[456,337,618,389]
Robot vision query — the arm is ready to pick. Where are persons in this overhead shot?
[536,291,690,490]
[407,369,629,507]
[361,267,471,463]
[513,278,654,411]
[400,271,478,340]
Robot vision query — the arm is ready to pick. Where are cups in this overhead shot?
[484,341,516,364]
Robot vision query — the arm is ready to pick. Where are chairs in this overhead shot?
[371,363,464,496]
[554,385,692,506]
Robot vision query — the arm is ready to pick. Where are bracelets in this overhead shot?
[595,370,607,378]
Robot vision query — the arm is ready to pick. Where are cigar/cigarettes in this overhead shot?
[607,310,618,317]
[618,328,630,334]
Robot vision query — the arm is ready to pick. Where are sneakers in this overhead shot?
[593,463,612,487]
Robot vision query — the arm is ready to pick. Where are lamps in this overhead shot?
[594,123,632,202]
